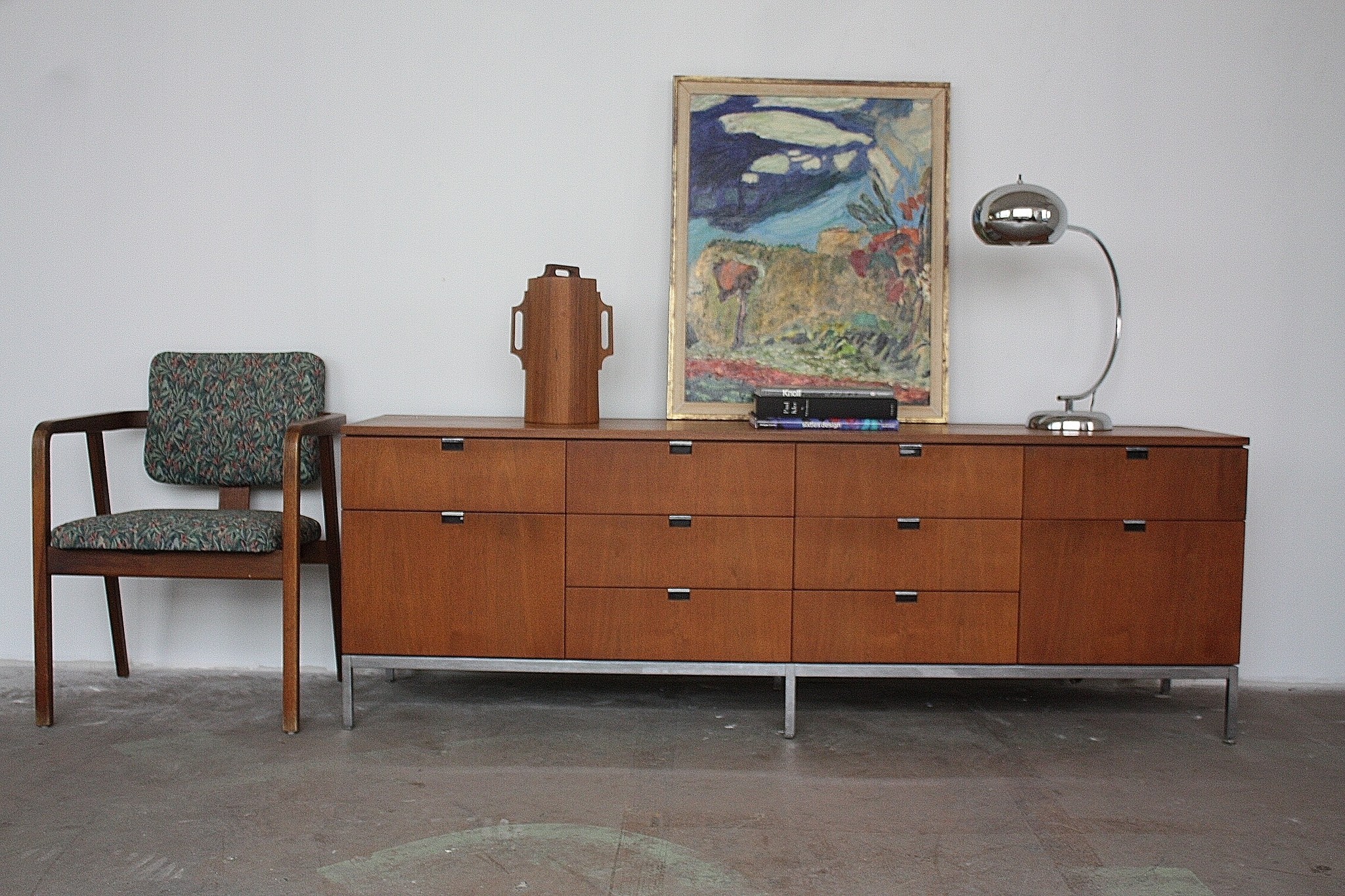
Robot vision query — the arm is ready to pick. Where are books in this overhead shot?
[748,387,900,433]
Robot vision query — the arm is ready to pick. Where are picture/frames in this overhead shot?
[667,79,950,423]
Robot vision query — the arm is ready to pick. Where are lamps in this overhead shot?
[972,170,1125,430]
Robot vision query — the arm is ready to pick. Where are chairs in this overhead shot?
[33,351,341,732]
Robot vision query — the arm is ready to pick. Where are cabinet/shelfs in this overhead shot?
[328,413,1252,724]
